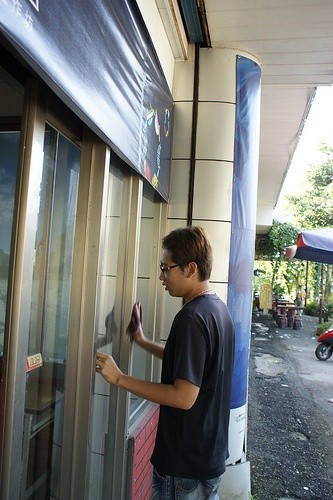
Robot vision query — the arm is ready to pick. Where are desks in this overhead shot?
[272,301,294,306]
[277,305,306,327]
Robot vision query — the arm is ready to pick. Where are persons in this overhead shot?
[96,226,234,500]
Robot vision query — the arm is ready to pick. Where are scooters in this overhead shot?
[314,328,333,361]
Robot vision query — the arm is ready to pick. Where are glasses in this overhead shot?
[160,261,179,273]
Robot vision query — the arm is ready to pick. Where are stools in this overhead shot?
[273,301,302,330]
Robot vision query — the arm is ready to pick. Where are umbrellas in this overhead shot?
[286,228,333,264]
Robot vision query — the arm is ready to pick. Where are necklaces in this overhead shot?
[182,289,212,309]
